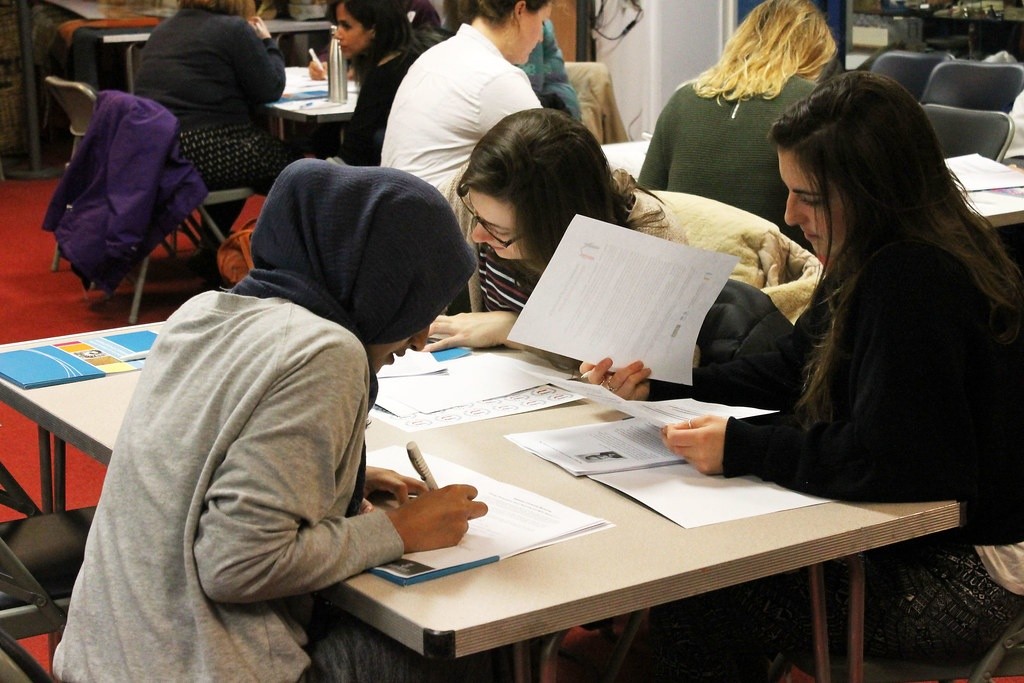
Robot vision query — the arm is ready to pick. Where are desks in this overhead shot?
[57,14,332,96]
[263,66,361,161]
[933,0,1024,61]
[599,137,1024,231]
[0,319,967,683]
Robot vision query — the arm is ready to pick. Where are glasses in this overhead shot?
[460,183,525,249]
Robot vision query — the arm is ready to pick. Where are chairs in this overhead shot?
[1,462,97,639]
[126,41,145,94]
[46,74,186,288]
[871,49,1024,164]
[768,593,1024,683]
[93,89,254,324]
[636,190,779,291]
[0,536,68,683]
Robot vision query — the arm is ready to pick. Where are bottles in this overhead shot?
[328,26,348,102]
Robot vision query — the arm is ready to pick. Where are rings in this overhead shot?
[599,376,604,386]
[689,419,692,429]
[606,381,620,391]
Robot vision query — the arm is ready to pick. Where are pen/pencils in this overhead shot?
[309,48,327,79]
[406,441,438,490]
[581,370,591,379]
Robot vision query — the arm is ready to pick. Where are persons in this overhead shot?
[52,156,487,683]
[636,1,839,256]
[580,70,1024,683]
[427,108,684,355]
[135,0,583,276]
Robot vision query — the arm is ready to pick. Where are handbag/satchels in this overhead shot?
[217,229,257,284]
